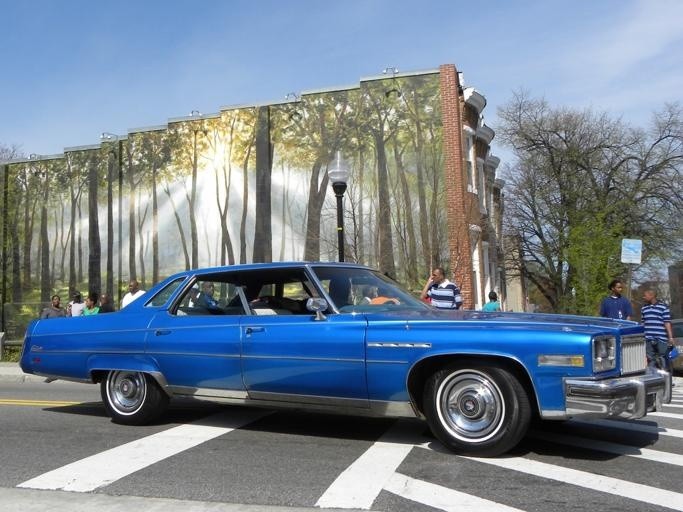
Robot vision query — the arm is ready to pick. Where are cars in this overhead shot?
[19,263,670,458]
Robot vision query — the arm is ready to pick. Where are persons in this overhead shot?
[357,285,374,305]
[420,267,464,310]
[64,291,88,316]
[192,282,219,308]
[40,294,67,318]
[639,287,676,388]
[598,279,633,322]
[178,283,204,308]
[122,280,152,308]
[98,294,115,312]
[481,291,513,313]
[82,292,103,315]
[250,276,353,313]
[371,286,400,305]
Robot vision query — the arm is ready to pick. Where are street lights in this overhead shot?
[328,150,351,264]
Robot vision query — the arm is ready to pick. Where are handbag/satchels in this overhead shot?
[665,344,683,377]
